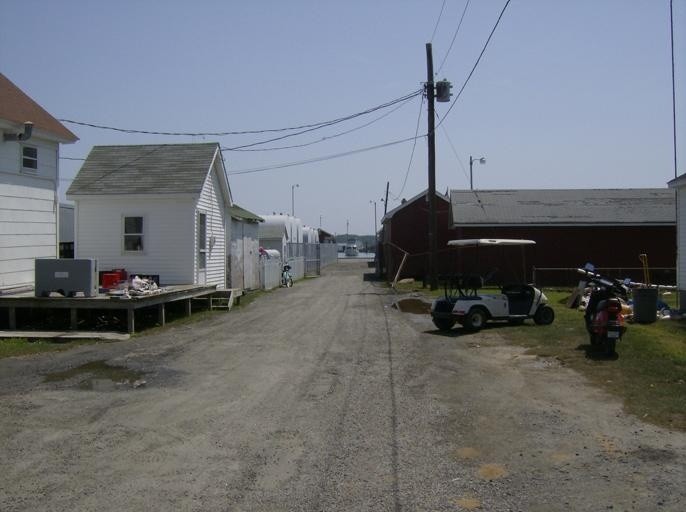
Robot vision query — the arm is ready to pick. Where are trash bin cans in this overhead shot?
[632,288,658,323]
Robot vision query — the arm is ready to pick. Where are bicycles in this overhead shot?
[281,264,294,289]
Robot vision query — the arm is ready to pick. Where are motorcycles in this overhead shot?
[582,269,627,352]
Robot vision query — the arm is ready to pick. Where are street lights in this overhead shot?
[468,155,487,190]
[319,214,323,228]
[343,215,352,241]
[369,199,378,243]
[290,183,300,216]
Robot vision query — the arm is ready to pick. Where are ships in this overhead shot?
[343,242,359,256]
